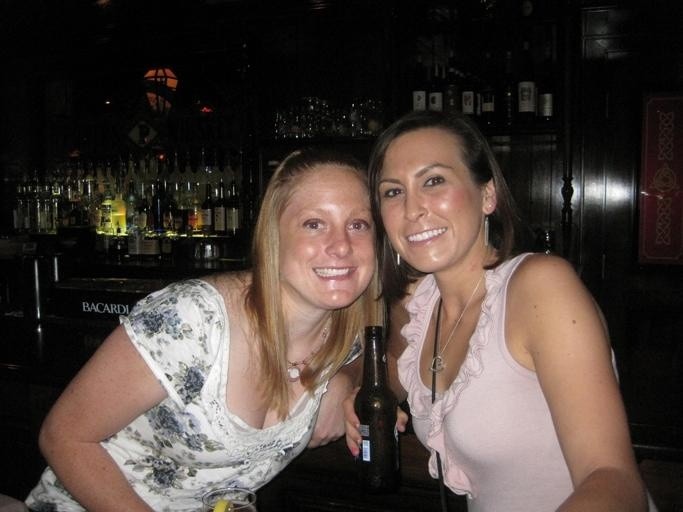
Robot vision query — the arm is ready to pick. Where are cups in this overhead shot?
[199,486,258,512]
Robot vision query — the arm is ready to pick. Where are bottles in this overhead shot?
[350,323,404,498]
[410,53,562,129]
[7,153,242,239]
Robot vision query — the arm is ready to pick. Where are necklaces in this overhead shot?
[428,275,480,373]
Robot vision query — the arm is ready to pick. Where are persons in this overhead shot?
[339,110,660,511]
[20,144,391,512]
[285,317,335,384]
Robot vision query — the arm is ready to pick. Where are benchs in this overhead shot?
[251,410,468,512]
